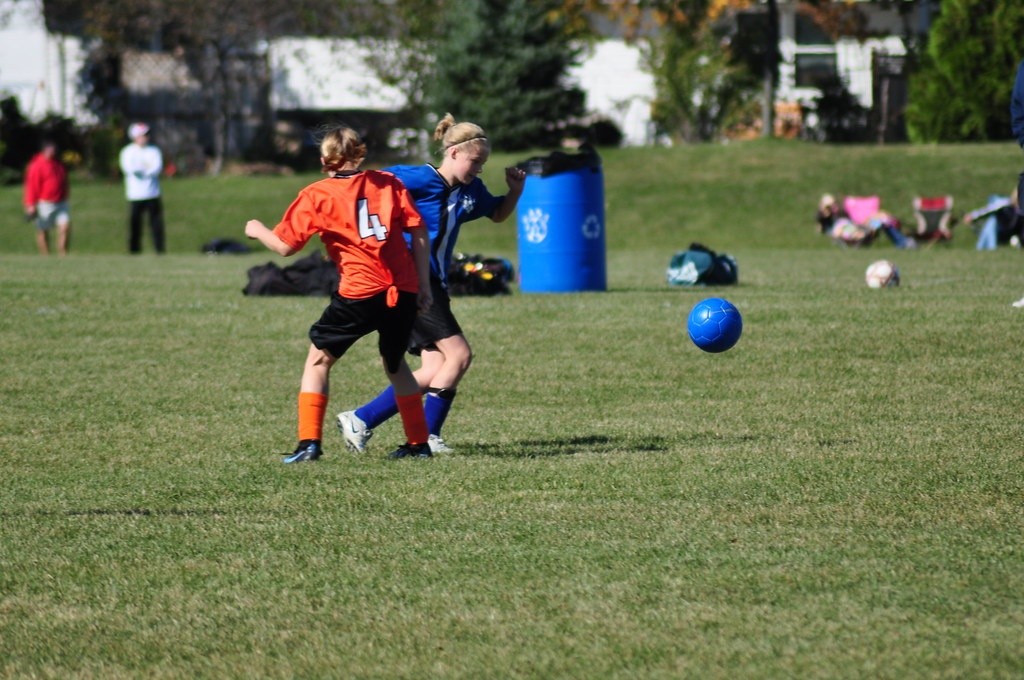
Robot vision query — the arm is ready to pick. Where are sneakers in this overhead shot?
[428,434,455,457]
[388,441,433,459]
[336,409,373,456]
[278,445,322,462]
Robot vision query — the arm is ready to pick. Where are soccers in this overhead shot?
[689,297,743,352]
[867,258,900,289]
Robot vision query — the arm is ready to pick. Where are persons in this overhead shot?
[818,194,915,253]
[962,203,1017,252]
[118,121,167,255]
[332,110,526,457]
[243,126,435,462]
[24,143,70,257]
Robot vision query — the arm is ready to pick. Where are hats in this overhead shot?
[128,123,150,140]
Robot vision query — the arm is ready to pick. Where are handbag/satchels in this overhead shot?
[666,243,738,288]
